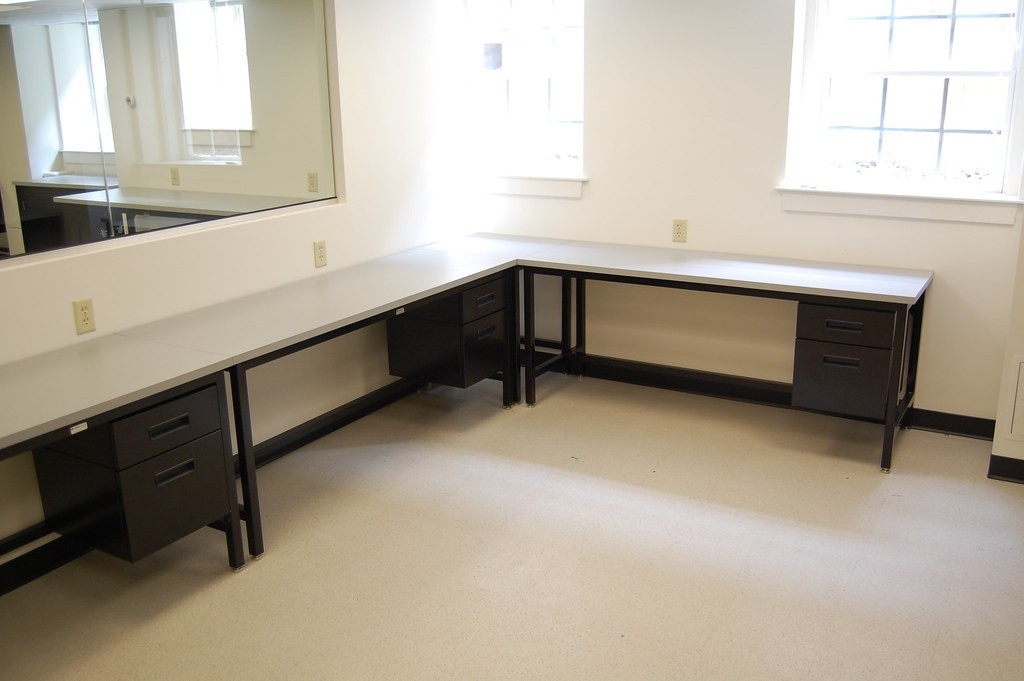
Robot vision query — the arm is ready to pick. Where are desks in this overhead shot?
[0,231,935,572]
[11,178,309,253]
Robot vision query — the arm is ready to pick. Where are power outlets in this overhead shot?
[72,298,96,334]
[314,240,326,268]
[170,167,180,185]
[307,172,319,192]
[672,219,687,243]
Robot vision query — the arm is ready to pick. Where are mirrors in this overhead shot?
[0,0,347,273]
